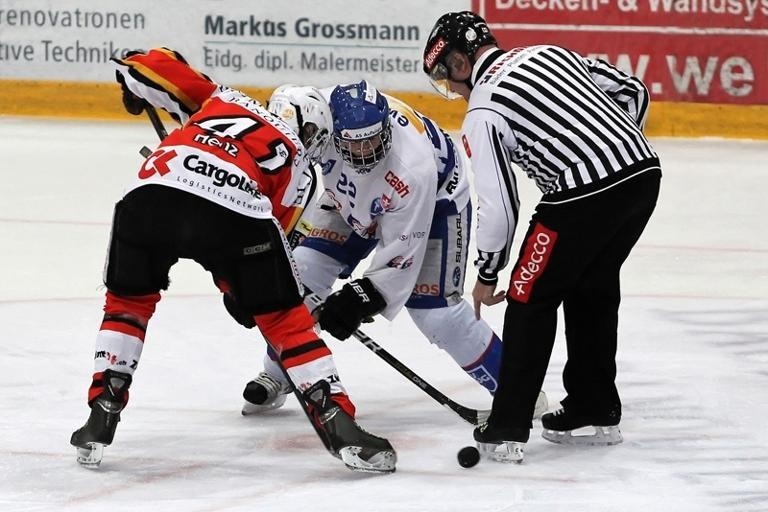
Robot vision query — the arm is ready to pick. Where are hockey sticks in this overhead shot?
[303,283,549,425]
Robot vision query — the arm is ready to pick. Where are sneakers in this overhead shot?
[473,415,530,445]
[541,397,622,431]
[243,371,294,405]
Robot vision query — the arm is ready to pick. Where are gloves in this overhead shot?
[113,68,152,115]
[222,291,259,330]
[318,276,387,341]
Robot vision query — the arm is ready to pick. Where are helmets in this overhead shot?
[422,10,494,80]
[267,83,334,155]
[329,79,392,170]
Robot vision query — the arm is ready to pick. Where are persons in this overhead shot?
[240,78,551,421]
[65,47,398,466]
[420,9,664,445]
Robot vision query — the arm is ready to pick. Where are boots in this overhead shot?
[70,369,132,451]
[301,380,397,461]
